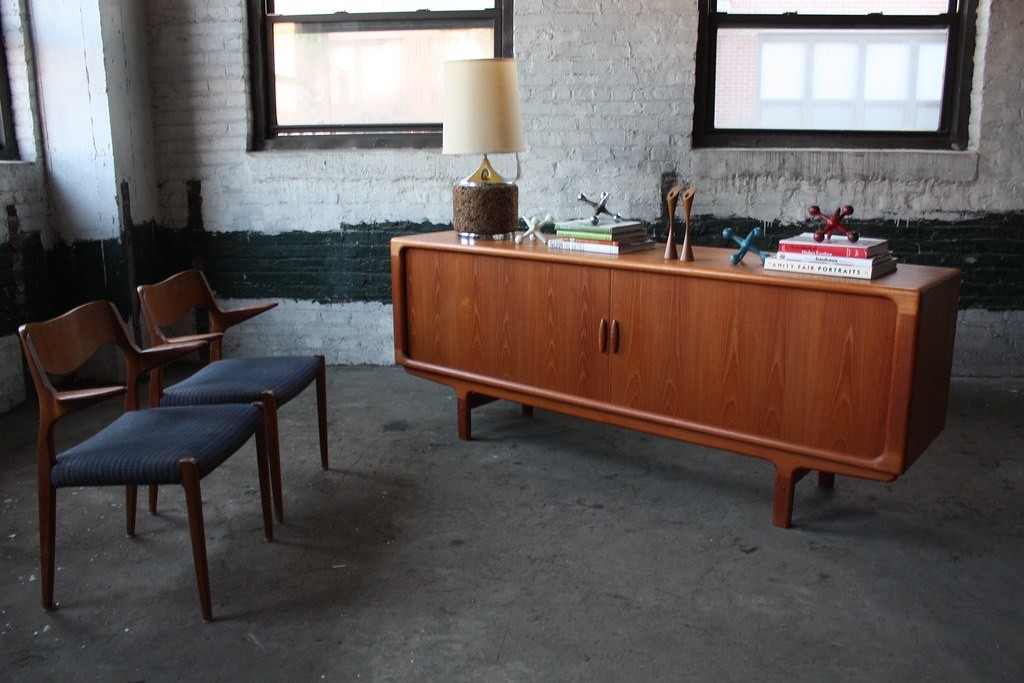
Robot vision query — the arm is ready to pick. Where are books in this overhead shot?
[763,231,898,280]
[547,215,656,254]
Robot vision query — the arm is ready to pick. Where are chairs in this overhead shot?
[137,269,329,525]
[19,300,273,624]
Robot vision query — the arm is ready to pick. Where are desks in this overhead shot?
[391,230,961,530]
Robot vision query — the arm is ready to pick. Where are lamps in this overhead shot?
[442,58,527,239]
